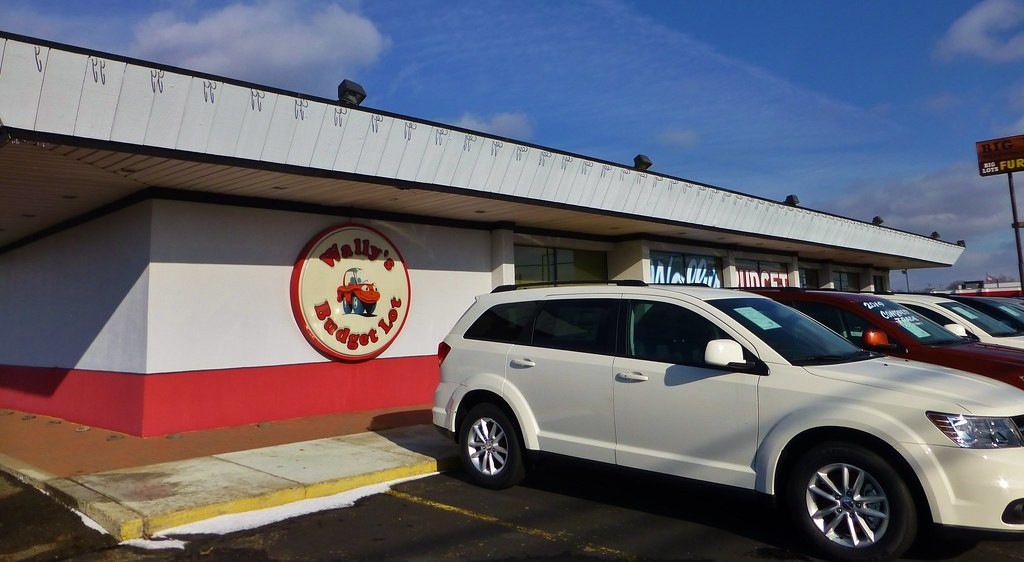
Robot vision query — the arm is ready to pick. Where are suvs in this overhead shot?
[431,279,1024,562]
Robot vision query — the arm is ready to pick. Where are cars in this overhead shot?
[723,287,1024,392]
